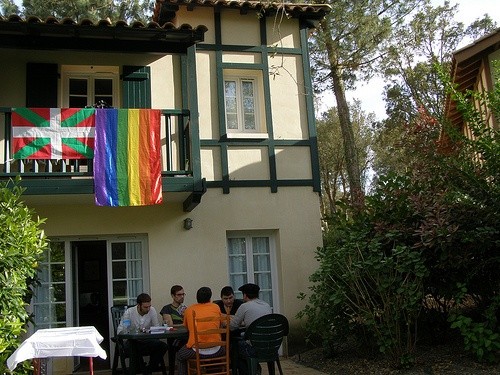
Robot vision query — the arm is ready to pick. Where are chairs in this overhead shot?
[229,314,289,375]
[188,311,230,375]
[111,306,167,375]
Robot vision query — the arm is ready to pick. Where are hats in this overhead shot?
[238,283,260,291]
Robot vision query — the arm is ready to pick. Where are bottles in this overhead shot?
[120,305,131,333]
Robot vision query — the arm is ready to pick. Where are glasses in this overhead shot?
[177,293,184,296]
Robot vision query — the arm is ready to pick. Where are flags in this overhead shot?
[10,108,95,159]
[95,109,163,206]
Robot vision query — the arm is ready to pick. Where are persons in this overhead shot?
[117,293,168,375]
[222,283,272,375]
[215,286,246,340]
[174,286,224,375]
[160,285,188,375]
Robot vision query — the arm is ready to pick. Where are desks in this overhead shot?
[7,326,107,375]
[116,327,246,375]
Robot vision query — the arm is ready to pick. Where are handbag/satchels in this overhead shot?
[239,361,262,375]
